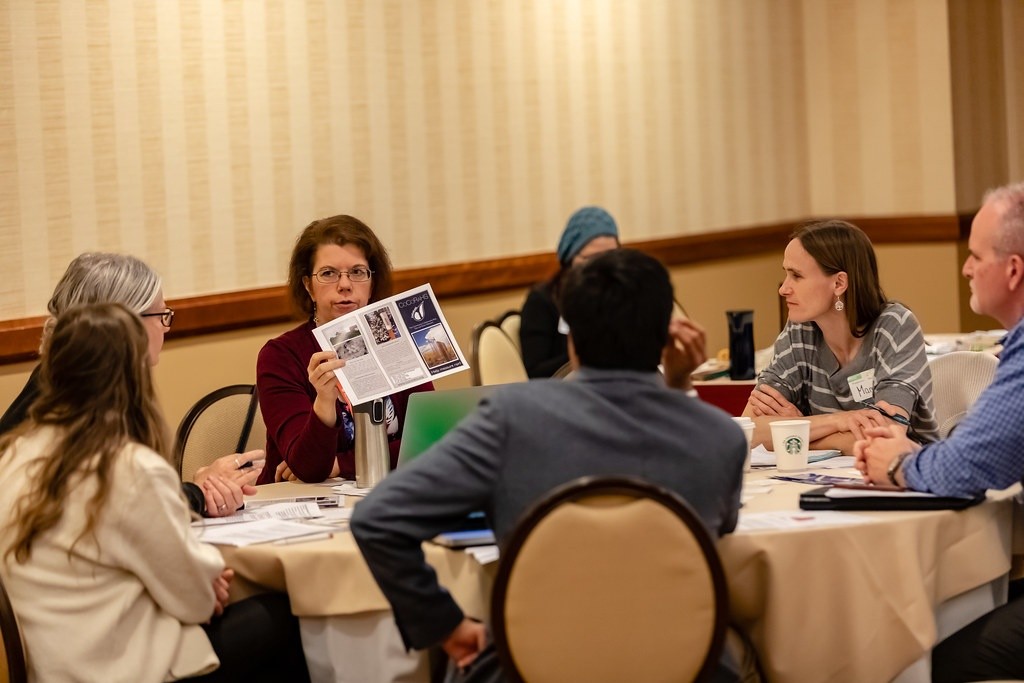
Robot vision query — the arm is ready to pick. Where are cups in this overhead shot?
[731,416,755,470]
[768,419,813,472]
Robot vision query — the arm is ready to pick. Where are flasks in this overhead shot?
[724,309,756,381]
[352,397,391,488]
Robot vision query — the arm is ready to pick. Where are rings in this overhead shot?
[241,469,244,474]
[235,459,241,468]
[217,504,226,509]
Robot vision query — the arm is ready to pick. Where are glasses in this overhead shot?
[311,264,375,283]
[141,307,175,327]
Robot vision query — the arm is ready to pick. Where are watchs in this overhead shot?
[886,452,911,485]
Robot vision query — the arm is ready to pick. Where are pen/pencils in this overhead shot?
[239,461,253,469]
[836,483,906,492]
[862,401,911,426]
[335,384,350,405]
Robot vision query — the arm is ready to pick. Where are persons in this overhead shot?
[256,215,436,485]
[0,251,307,683]
[518,206,623,381]
[350,250,748,683]
[854,183,1024,683]
[741,220,940,458]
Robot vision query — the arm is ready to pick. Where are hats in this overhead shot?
[557,206,618,272]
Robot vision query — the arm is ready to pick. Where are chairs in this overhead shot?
[928,351,1001,441]
[470,299,689,386]
[489,475,741,683]
[171,384,258,483]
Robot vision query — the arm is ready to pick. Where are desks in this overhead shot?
[691,333,1004,417]
[187,468,1024,683]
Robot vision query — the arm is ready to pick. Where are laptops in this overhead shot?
[396,380,506,545]
